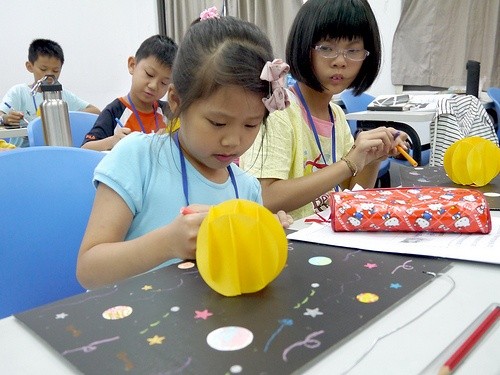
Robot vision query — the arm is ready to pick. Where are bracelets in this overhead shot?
[341,156,358,177]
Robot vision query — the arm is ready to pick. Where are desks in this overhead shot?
[0,185,500,375]
[345,97,495,166]
[0,124,28,138]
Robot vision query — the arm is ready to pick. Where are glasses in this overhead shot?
[313,43,370,61]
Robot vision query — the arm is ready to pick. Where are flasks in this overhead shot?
[29,75,73,147]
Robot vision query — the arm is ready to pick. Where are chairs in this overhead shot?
[0,146,109,317]
[342,89,429,164]
[26,110,99,150]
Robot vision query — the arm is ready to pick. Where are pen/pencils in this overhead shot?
[394,131,400,140]
[397,145,417,167]
[439,306,500,375]
[115,118,124,127]
[4,101,28,123]
[179,206,197,214]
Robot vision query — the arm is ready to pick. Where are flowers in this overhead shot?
[198,5,220,20]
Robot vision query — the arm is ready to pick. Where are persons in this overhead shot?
[0,39,101,148]
[77,5,297,293]
[239,0,407,222]
[80,34,178,151]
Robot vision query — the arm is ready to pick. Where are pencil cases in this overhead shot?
[329,186,492,235]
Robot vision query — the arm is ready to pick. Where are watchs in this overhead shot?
[0,114,5,124]
